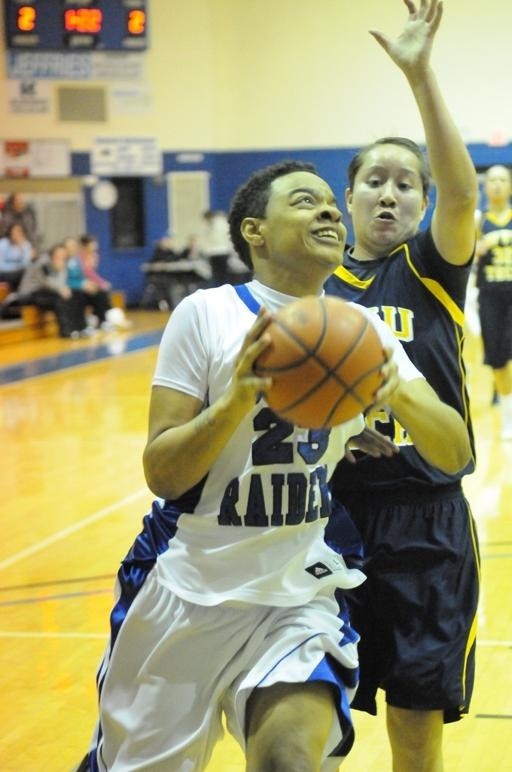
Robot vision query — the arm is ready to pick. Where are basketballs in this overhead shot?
[254,296,385,428]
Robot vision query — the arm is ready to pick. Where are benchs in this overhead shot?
[0,279,126,332]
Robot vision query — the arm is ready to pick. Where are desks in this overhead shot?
[138,237,222,313]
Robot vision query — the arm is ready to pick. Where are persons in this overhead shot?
[2,189,247,343]
[461,163,512,446]
[74,161,475,772]
[320,0,479,771]
